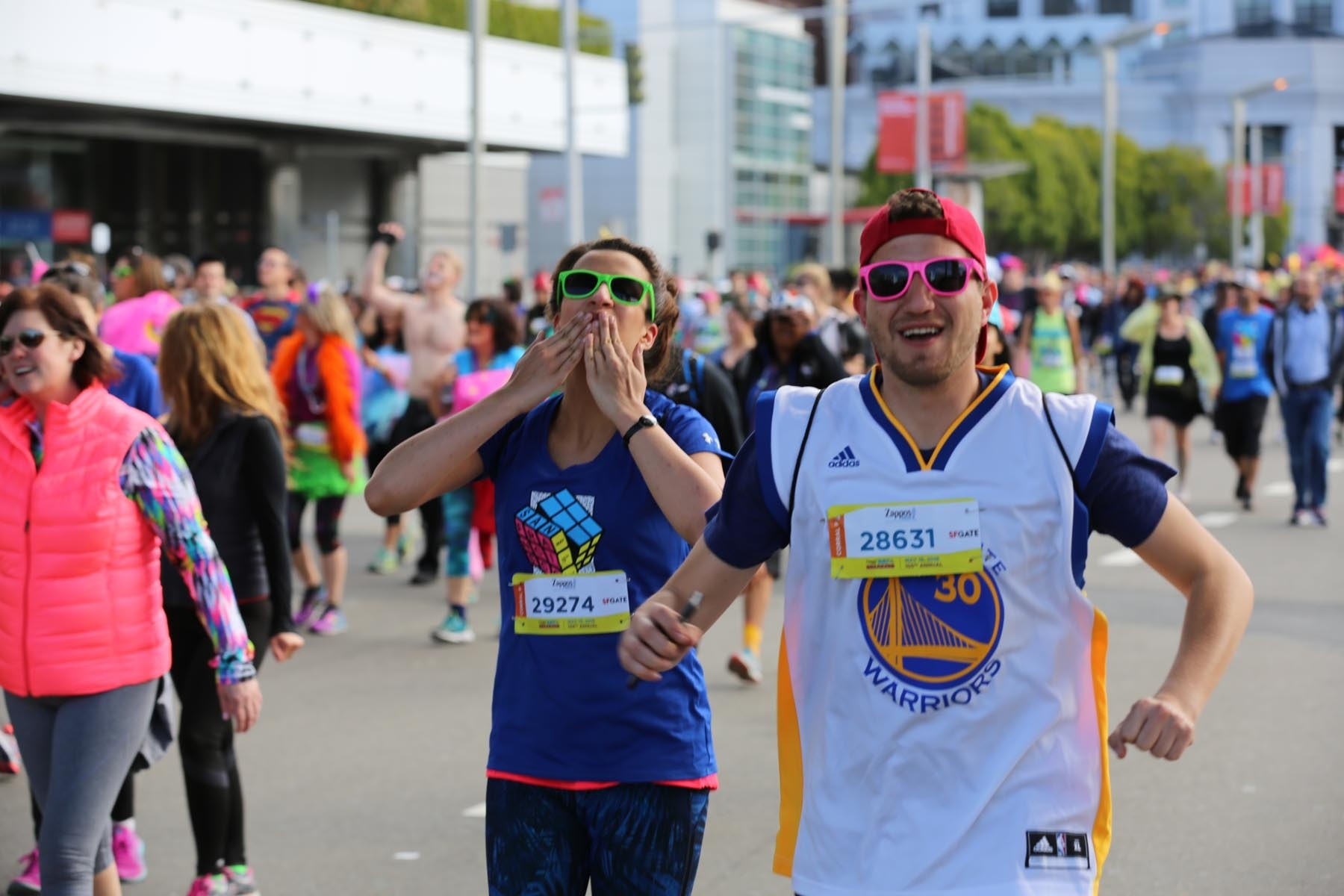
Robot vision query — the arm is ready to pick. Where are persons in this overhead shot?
[622,187,1254,896]
[984,248,1343,525]
[0,220,875,896]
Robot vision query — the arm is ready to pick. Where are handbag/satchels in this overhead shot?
[285,423,369,497]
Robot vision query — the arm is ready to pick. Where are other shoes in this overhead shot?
[293,586,325,628]
[0,723,22,775]
[432,611,476,643]
[728,648,763,685]
[468,581,481,603]
[367,531,412,575]
[411,570,434,585]
[1176,473,1327,529]
[310,606,347,636]
[187,864,260,896]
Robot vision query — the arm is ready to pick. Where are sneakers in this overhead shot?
[8,850,42,896]
[111,824,147,884]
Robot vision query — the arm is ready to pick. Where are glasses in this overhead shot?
[41,261,91,284]
[859,256,986,302]
[556,270,656,323]
[466,304,492,325]
[111,266,134,280]
[0,329,64,357]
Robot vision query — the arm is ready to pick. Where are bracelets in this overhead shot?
[622,415,658,448]
[371,231,398,246]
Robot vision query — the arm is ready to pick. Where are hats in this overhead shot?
[988,246,1336,302]
[858,189,986,271]
[682,261,857,338]
